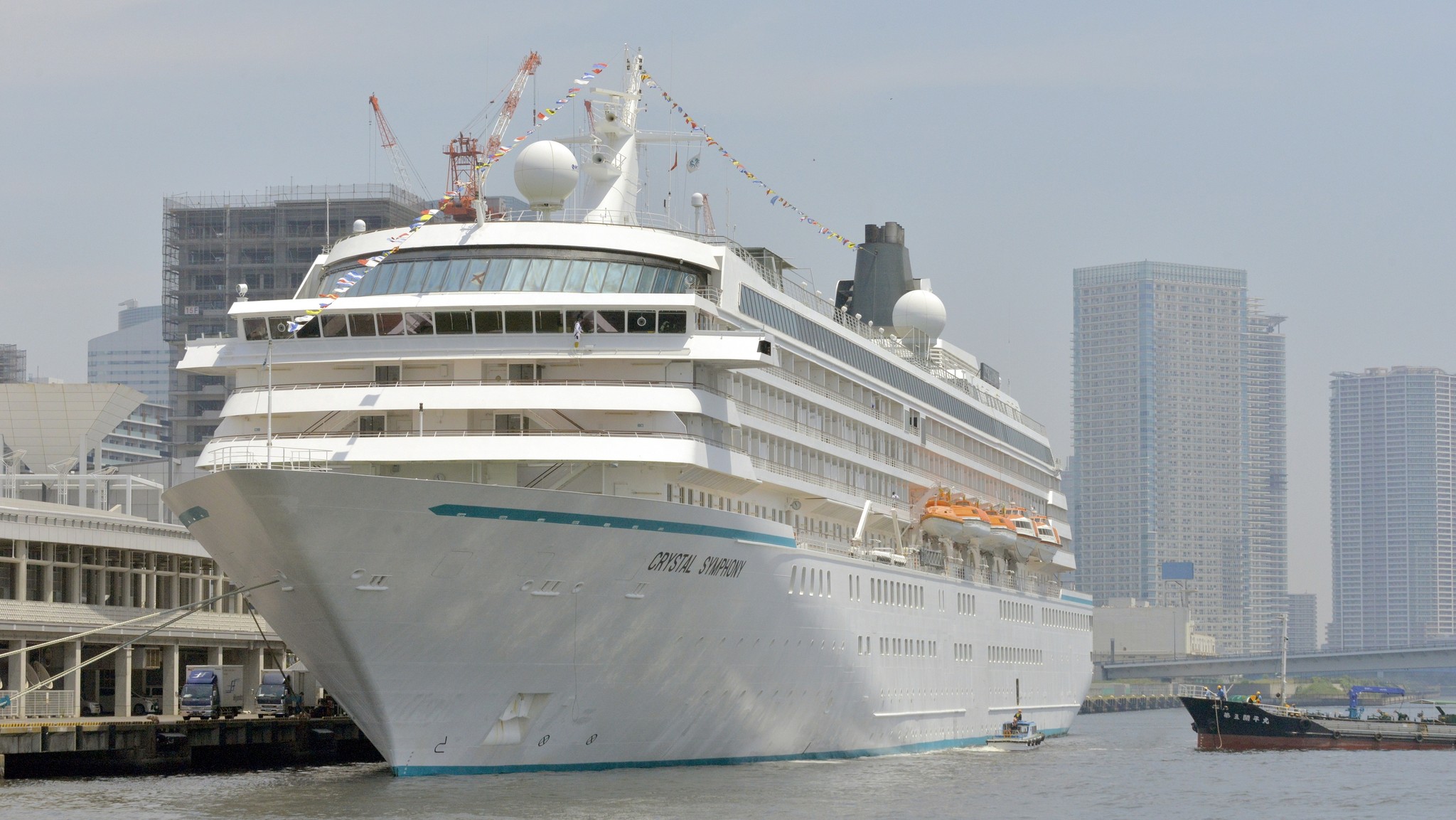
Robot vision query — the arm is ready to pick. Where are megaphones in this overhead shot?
[605,113,616,123]
[592,153,604,165]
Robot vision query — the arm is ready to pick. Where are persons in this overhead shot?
[1253,691,1262,703]
[1204,686,1211,699]
[1215,685,1225,701]
[573,318,587,348]
[1014,709,1022,721]
[1011,718,1018,730]
[576,311,584,322]
[296,692,304,720]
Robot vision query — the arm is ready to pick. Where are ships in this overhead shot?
[162,43,1099,778]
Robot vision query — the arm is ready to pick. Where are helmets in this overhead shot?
[1256,691,1260,695]
[1204,686,1208,690]
[1217,685,1221,689]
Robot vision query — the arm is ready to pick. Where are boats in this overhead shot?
[1175,684,1455,750]
[920,486,1063,564]
[986,718,1047,751]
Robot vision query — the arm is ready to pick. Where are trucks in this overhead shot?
[179,665,244,720]
[254,668,324,719]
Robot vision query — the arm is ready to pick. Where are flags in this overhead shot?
[641,70,863,251]
[286,62,610,333]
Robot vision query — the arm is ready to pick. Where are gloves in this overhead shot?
[1204,694,1206,696]
[1214,693,1217,697]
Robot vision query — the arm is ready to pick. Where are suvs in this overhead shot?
[79,685,159,716]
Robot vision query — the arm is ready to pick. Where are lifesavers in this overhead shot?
[1374,733,1383,742]
[1297,718,1312,731]
[1415,734,1424,743]
[1333,730,1341,739]
